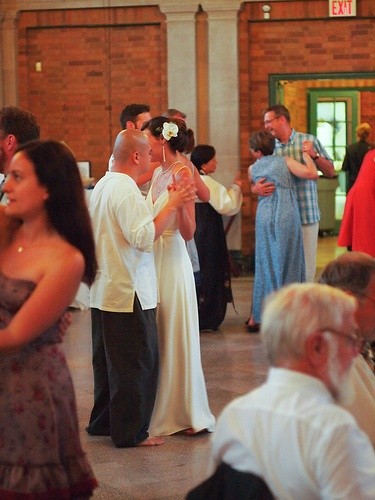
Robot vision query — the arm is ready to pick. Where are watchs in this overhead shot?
[312,152,320,160]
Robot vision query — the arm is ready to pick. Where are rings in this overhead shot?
[304,145,307,148]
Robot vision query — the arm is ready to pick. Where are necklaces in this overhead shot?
[17,246,25,253]
[155,160,182,194]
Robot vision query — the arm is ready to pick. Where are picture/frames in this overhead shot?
[77,161,90,179]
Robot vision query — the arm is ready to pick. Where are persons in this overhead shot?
[86,129,198,448]
[136,119,210,203]
[109,104,151,171]
[248,131,319,330]
[251,104,334,282]
[319,252,375,447]
[140,118,216,437]
[208,284,375,500]
[0,106,39,204]
[190,145,246,332]
[0,140,98,500]
[342,123,373,195]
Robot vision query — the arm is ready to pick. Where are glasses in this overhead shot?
[319,327,366,356]
[264,116,280,125]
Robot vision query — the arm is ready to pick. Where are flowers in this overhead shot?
[162,122,179,141]
